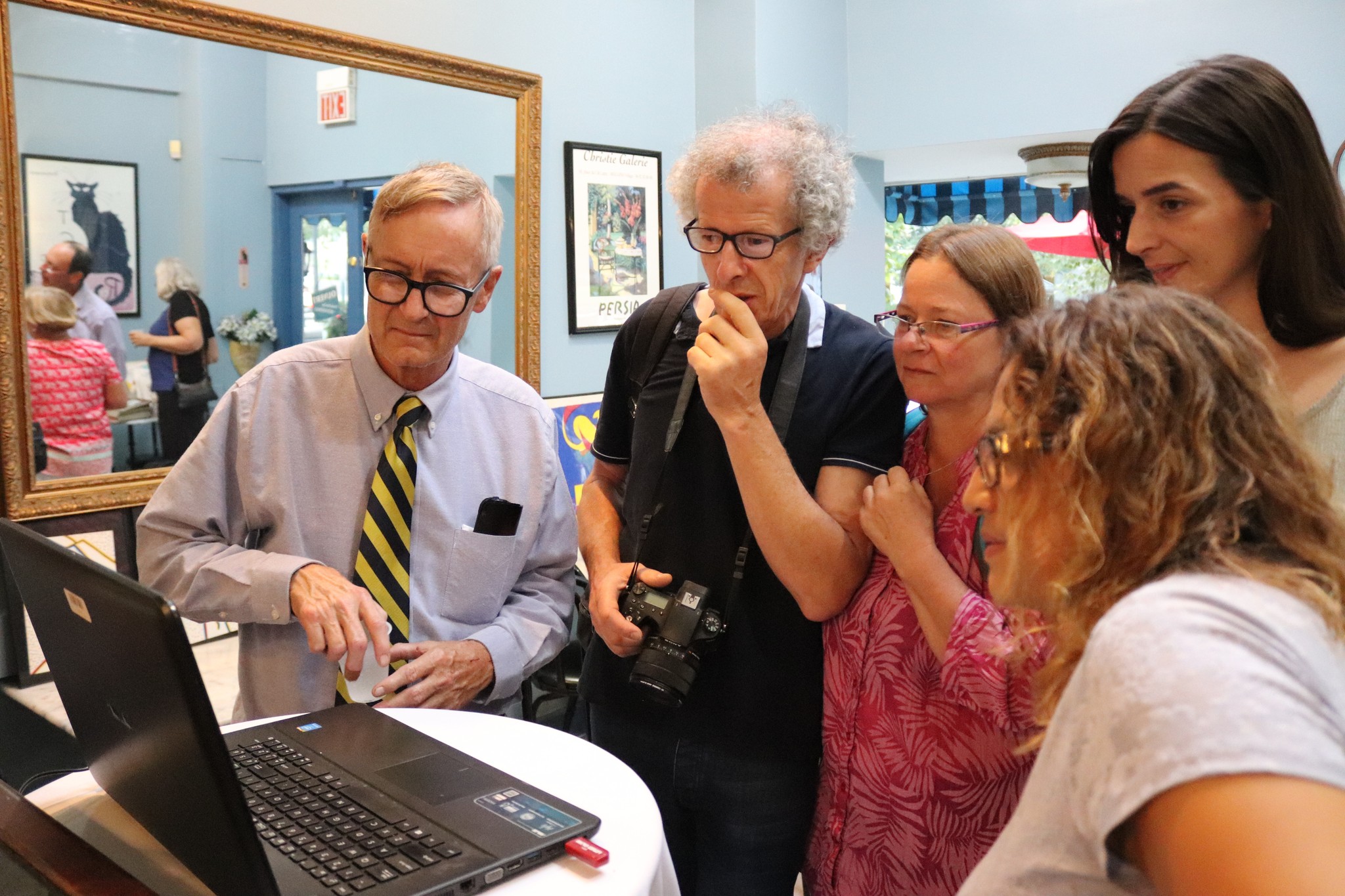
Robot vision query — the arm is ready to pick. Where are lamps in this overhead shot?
[1017,142,1094,202]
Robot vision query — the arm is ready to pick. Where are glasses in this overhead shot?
[873,309,1001,344]
[971,430,1072,488]
[362,244,491,318]
[683,216,802,260]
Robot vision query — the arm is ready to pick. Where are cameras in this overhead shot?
[620,581,725,709]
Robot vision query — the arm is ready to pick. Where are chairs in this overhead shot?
[122,360,160,470]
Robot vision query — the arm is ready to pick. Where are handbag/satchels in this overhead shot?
[176,376,218,409]
[31,421,47,473]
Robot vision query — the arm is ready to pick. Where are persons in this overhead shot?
[593,54,1345,896]
[135,166,580,713]
[24,237,218,486]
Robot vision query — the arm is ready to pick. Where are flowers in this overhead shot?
[216,308,279,345]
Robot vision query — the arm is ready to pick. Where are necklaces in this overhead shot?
[911,426,962,481]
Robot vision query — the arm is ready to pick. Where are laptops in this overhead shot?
[0,519,602,896]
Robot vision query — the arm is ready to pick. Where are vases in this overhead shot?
[230,341,262,377]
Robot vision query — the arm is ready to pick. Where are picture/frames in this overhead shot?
[564,141,664,336]
[21,153,141,317]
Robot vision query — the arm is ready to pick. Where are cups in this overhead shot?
[130,328,143,348]
[338,619,393,704]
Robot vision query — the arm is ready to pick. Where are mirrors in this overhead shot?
[0,0,542,521]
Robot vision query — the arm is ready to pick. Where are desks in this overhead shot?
[0,707,679,896]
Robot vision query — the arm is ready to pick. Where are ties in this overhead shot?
[333,391,429,707]
